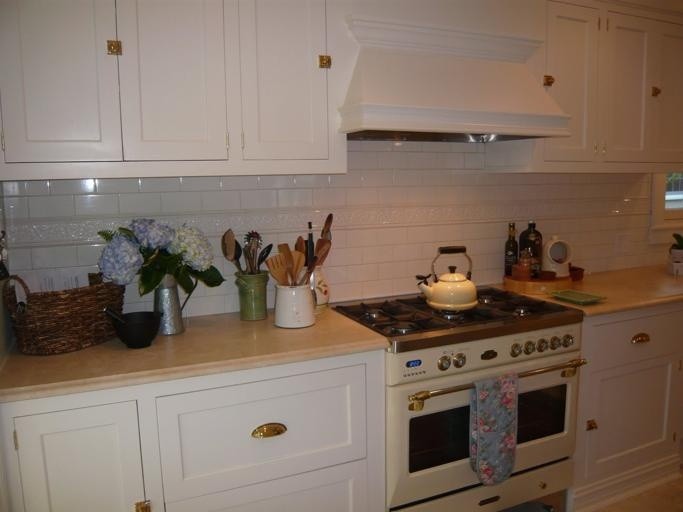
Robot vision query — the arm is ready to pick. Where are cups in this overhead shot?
[235,272,268,321]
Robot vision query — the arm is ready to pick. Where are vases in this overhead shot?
[147,274,190,335]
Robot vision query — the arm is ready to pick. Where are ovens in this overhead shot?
[378,348,581,512]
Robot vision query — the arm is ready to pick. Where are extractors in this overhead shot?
[347,0,572,144]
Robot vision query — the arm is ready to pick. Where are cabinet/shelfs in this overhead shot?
[229,0,348,176]
[483,0,683,175]
[492,298,683,512]
[0,350,387,511]
[1,0,230,180]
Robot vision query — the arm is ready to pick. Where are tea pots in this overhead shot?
[416,246,478,311]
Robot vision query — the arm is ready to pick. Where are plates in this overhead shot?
[552,288,606,304]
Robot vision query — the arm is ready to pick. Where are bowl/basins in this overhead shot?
[109,312,159,349]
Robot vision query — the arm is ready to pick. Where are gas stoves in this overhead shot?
[333,286,582,388]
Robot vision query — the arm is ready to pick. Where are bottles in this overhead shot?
[274,285,316,330]
[299,264,330,307]
[503,221,544,280]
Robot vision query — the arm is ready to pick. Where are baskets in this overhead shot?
[0,274,126,357]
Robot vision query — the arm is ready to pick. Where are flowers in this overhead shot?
[82,213,226,304]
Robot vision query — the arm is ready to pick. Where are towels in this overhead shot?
[469,370,519,486]
